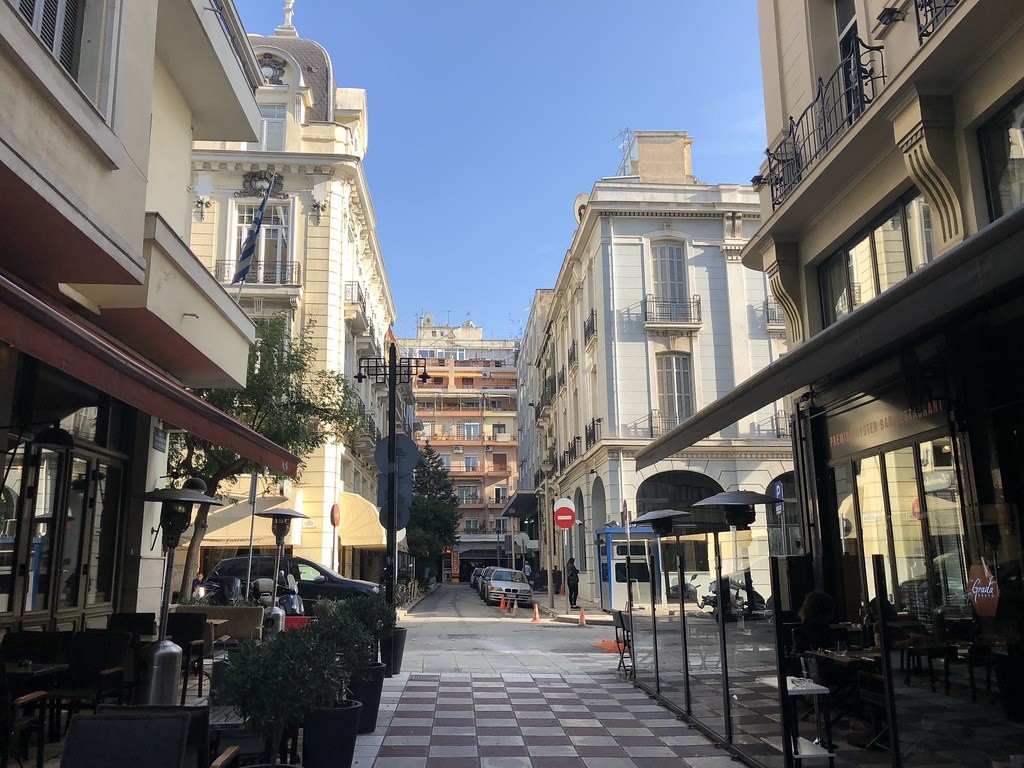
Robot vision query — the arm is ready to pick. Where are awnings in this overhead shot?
[0,274,303,477]
[181,490,301,547]
[634,201,1024,472]
[339,492,409,554]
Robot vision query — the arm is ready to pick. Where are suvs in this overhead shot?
[206,555,379,616]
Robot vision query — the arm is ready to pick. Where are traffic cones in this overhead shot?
[577,608,586,627]
[499,597,520,617]
[531,603,540,623]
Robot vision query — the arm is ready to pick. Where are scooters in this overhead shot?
[698,577,766,624]
[250,570,305,615]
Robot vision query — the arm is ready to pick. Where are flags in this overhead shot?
[230,178,273,285]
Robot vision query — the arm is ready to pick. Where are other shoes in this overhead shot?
[570,606,582,611]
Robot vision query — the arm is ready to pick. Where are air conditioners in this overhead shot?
[453,446,463,454]
[482,371,491,379]
[485,446,494,451]
[475,477,484,482]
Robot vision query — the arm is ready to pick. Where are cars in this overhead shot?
[470,567,534,608]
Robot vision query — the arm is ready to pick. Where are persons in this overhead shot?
[793,591,848,653]
[191,572,206,594]
[522,561,532,579]
[867,597,905,675]
[567,558,581,609]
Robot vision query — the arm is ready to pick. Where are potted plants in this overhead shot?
[224,590,407,768]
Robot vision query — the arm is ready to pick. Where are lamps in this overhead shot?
[0,417,74,493]
[751,176,770,185]
[876,7,905,25]
[160,470,207,491]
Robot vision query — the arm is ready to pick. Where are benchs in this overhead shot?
[177,604,264,659]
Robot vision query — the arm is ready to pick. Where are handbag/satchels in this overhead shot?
[568,576,578,587]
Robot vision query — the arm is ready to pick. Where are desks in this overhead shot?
[140,630,173,646]
[0,662,71,768]
[204,616,228,659]
[208,708,252,762]
[754,676,830,755]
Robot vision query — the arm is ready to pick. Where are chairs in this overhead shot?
[782,618,1001,739]
[613,608,723,680]
[0,612,207,768]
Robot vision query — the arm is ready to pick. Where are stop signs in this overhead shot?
[555,507,575,528]
[912,499,921,520]
[333,504,341,526]
